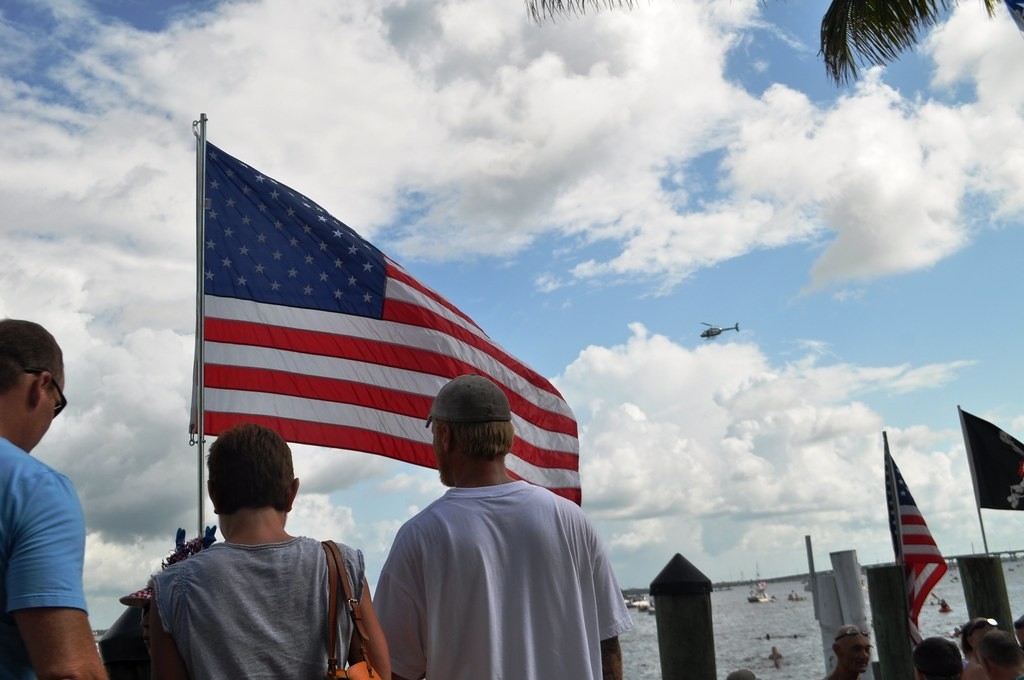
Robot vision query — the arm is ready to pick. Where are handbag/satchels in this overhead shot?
[321,540,384,680]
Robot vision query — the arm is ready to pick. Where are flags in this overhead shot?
[883,440,949,630]
[958,409,1024,512]
[187,129,583,507]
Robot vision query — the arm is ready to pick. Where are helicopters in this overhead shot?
[699,321,741,339]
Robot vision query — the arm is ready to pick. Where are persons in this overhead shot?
[0,319,109,679]
[373,373,634,680]
[119,422,392,680]
[822,616,1024,680]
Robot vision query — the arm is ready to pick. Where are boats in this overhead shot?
[747,569,776,603]
[624,593,655,616]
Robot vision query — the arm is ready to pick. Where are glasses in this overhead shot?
[835,628,869,641]
[24,366,67,418]
[969,618,999,636]
[1014,622,1024,629]
[140,605,149,620]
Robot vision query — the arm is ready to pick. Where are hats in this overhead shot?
[119,525,217,608]
[727,670,762,680]
[424,373,512,428]
